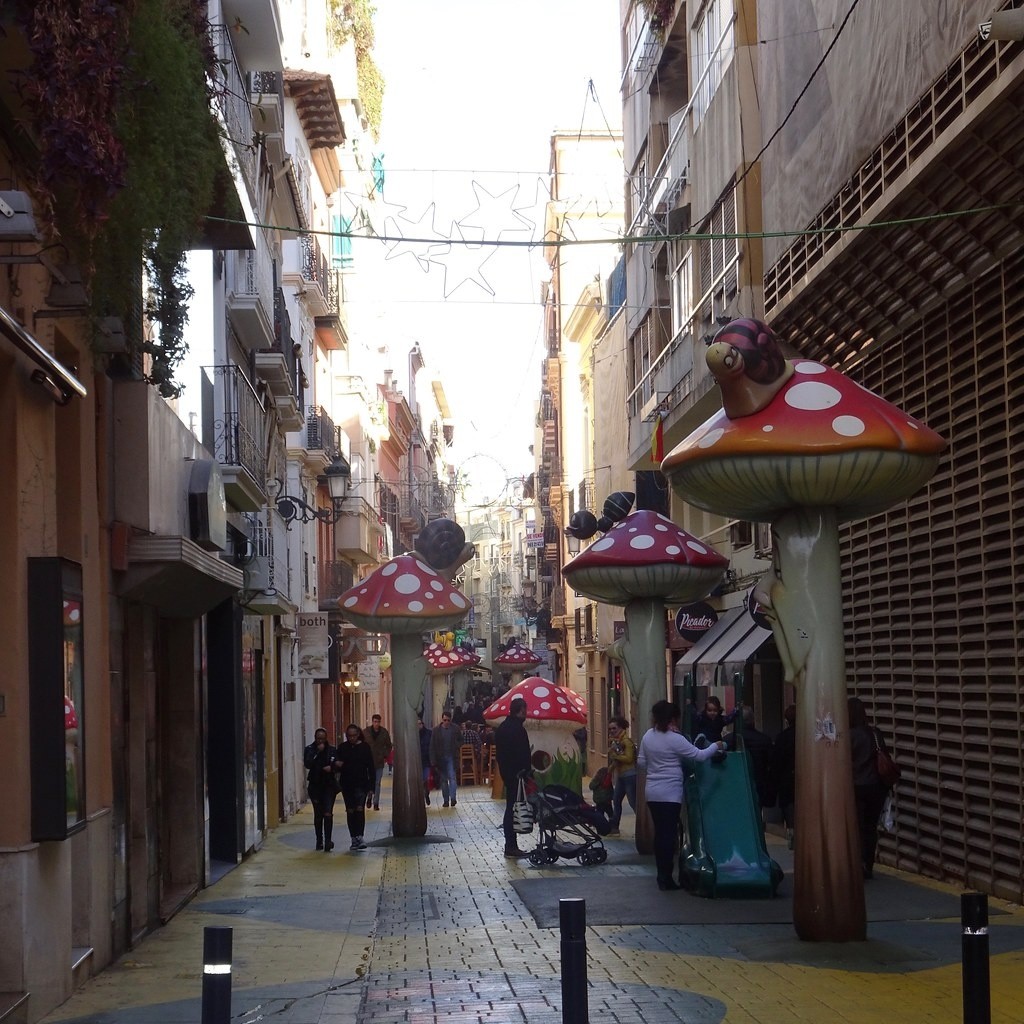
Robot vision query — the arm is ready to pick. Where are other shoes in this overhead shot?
[367,798,372,808]
[606,828,620,837]
[389,771,391,775]
[374,805,379,811]
[659,882,682,891]
[451,800,457,806]
[425,793,430,805]
[504,848,531,858]
[786,828,794,850]
[443,803,449,807]
[350,839,367,850]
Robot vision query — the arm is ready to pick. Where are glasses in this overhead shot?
[609,727,619,730]
[443,719,450,722]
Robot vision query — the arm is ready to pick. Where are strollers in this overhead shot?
[516,769,611,866]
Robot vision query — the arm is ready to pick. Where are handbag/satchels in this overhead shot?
[513,777,533,834]
[611,767,617,789]
[873,730,901,786]
[876,795,894,832]
[602,769,612,789]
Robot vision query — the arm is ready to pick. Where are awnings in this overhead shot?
[674,604,773,687]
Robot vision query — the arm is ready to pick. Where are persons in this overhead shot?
[686,695,743,744]
[742,704,796,849]
[304,728,337,851]
[496,699,531,858]
[417,717,432,805]
[451,685,506,751]
[604,716,637,837]
[362,714,392,811]
[847,697,890,880]
[428,712,464,808]
[589,767,614,818]
[335,724,376,850]
[637,700,722,890]
[386,744,394,775]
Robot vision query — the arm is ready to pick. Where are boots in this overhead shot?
[325,816,334,852]
[314,816,323,850]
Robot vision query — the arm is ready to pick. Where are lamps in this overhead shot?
[275,453,353,530]
